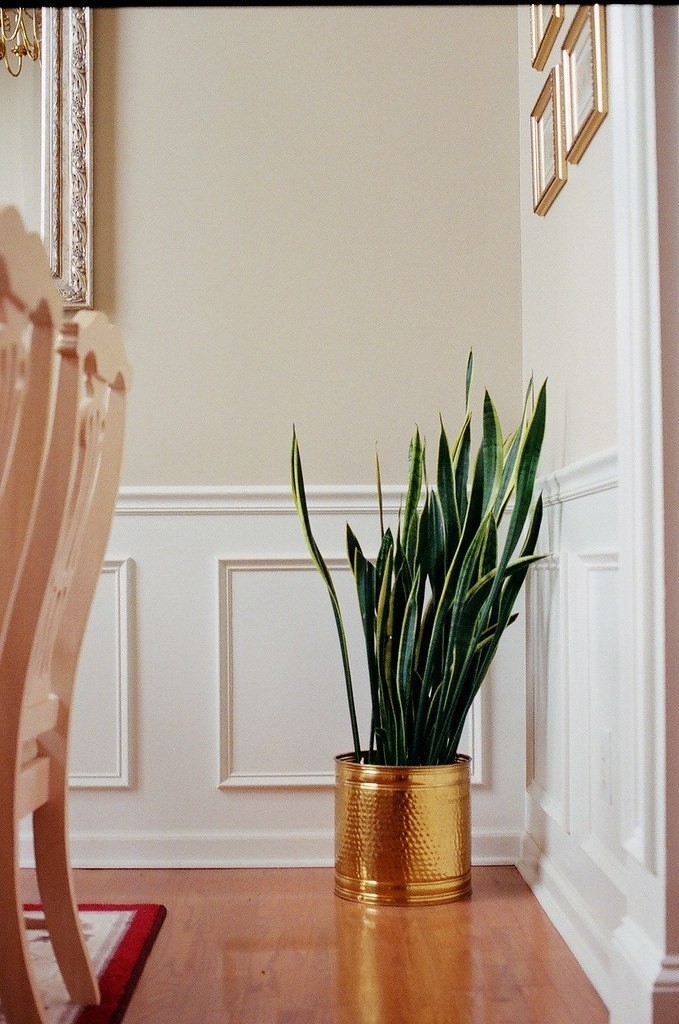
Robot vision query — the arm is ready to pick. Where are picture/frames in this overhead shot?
[529,4,565,71]
[560,5,609,165]
[529,65,569,217]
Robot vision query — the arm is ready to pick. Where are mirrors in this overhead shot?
[0,8,94,311]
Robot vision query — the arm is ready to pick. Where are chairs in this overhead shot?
[0,203,62,652]
[0,310,134,1024]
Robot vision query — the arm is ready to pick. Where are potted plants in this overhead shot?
[291,344,550,907]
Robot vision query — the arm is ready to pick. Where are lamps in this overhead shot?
[0,8,42,77]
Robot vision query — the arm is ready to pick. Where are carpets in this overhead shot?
[0,903,169,1024]
